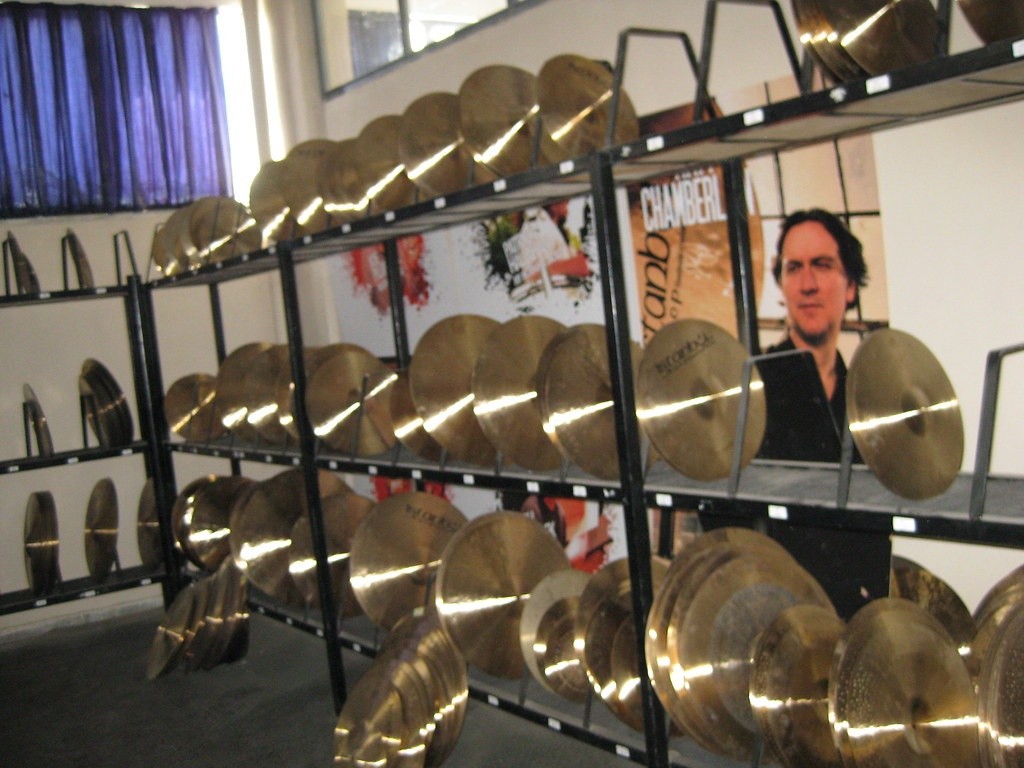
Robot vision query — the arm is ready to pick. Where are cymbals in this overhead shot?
[454,64,538,181]
[352,114,427,212]
[160,340,394,459]
[136,463,1024,768]
[409,313,502,460]
[313,138,386,225]
[535,53,641,157]
[20,470,162,595]
[473,316,571,473]
[3,195,261,299]
[527,76,569,163]
[635,319,768,482]
[398,91,493,195]
[75,355,135,447]
[391,363,442,456]
[791,0,1023,87]
[843,327,964,500]
[249,158,308,243]
[280,140,343,234]
[21,383,55,456]
[535,322,645,481]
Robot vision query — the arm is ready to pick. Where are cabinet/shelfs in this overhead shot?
[598,0,1024,768]
[0,229,176,622]
[141,149,662,768]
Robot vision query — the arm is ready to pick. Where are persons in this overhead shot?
[696,205,894,623]
